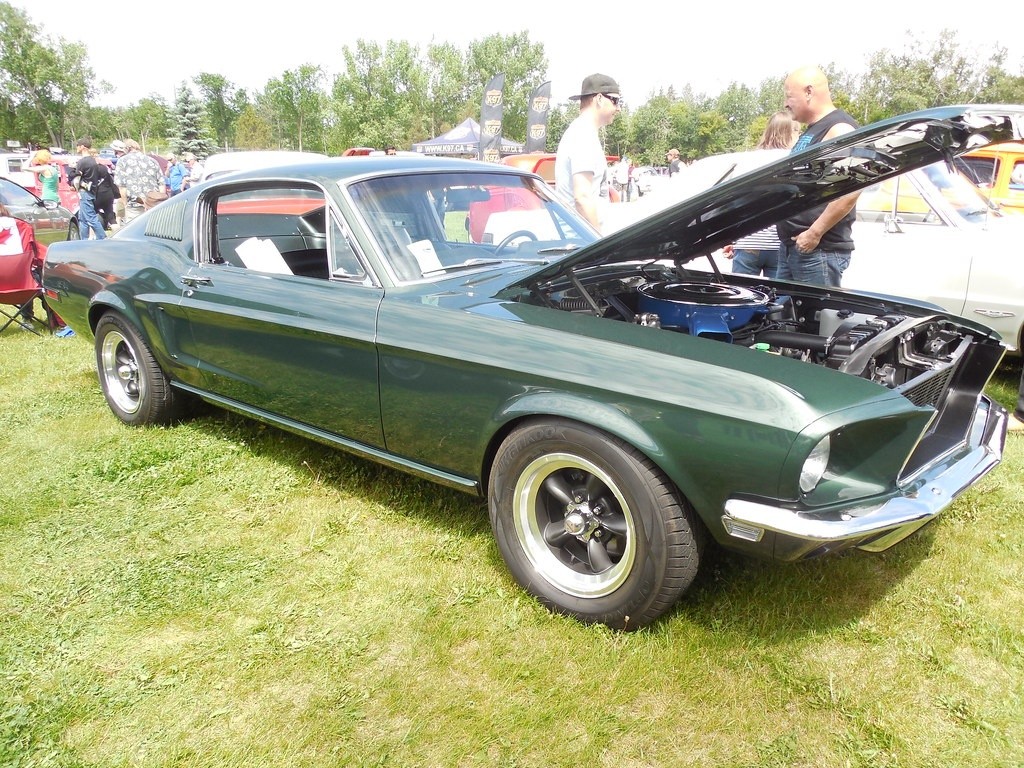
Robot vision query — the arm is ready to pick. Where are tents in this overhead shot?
[412,117,525,160]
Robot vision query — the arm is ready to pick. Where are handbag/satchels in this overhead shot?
[109,175,121,198]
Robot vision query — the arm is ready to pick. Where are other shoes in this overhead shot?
[98,178,104,186]
[21,318,33,329]
[85,181,93,192]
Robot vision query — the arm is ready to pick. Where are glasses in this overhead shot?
[594,93,619,105]
[185,160,191,163]
[387,153,396,155]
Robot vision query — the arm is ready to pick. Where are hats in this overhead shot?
[665,149,679,156]
[109,140,125,151]
[567,73,618,101]
[77,138,91,148]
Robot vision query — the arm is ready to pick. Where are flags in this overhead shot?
[479,73,505,162]
[527,81,551,154]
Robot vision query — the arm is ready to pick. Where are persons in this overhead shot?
[0,203,59,329]
[21,149,62,205]
[775,65,855,289]
[664,149,687,177]
[110,140,127,219]
[430,187,451,229]
[384,146,396,155]
[185,153,204,187]
[165,153,188,197]
[607,158,640,202]
[722,111,800,279]
[113,139,166,222]
[555,73,621,240]
[27,141,40,151]
[68,138,114,240]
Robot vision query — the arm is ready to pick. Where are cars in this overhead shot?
[26,103,1024,634]
[0,176,81,248]
[183,138,621,257]
[666,142,1024,362]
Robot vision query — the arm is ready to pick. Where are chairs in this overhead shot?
[0,215,57,338]
[275,248,357,281]
